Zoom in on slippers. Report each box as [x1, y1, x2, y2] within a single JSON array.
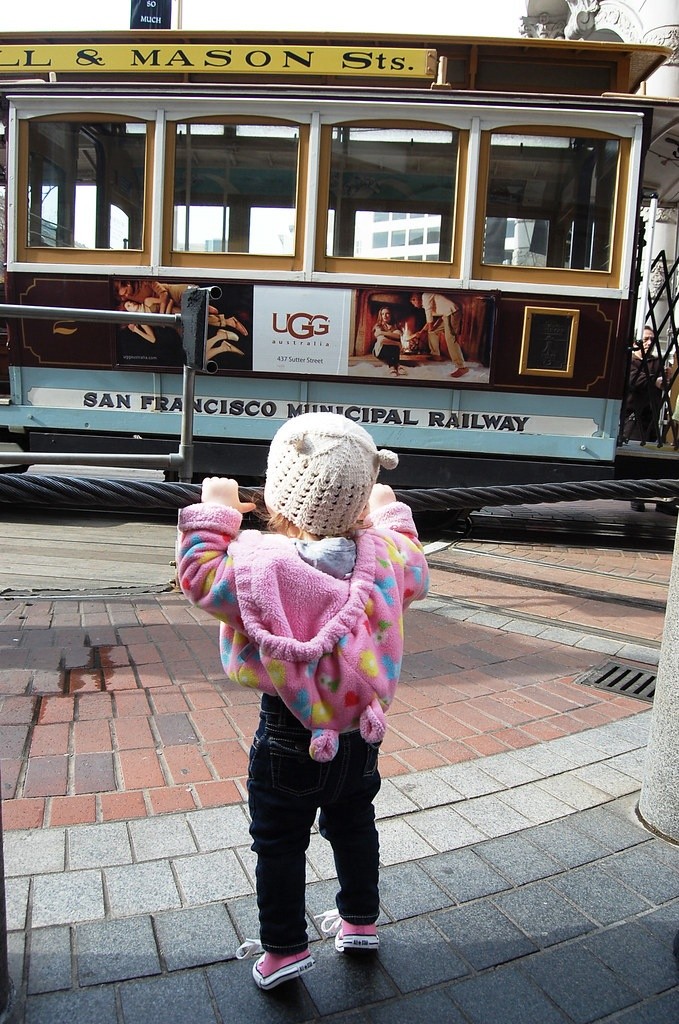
[[232, 317, 248, 336], [398, 365, 406, 375], [389, 369, 397, 377], [208, 305, 218, 314], [218, 329, 239, 341], [223, 340, 244, 356]]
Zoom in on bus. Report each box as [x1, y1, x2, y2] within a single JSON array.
[[0, 30, 679, 537]]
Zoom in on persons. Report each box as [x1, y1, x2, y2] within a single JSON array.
[[408, 290, 470, 379], [170, 407, 431, 992], [370, 304, 408, 377], [114, 279, 220, 317], [115, 295, 246, 357], [616, 324, 668, 444]]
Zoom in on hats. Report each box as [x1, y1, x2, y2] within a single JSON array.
[[264, 411, 399, 536]]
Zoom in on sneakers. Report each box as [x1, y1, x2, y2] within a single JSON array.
[[251, 949, 317, 990], [334, 918, 379, 954]]
